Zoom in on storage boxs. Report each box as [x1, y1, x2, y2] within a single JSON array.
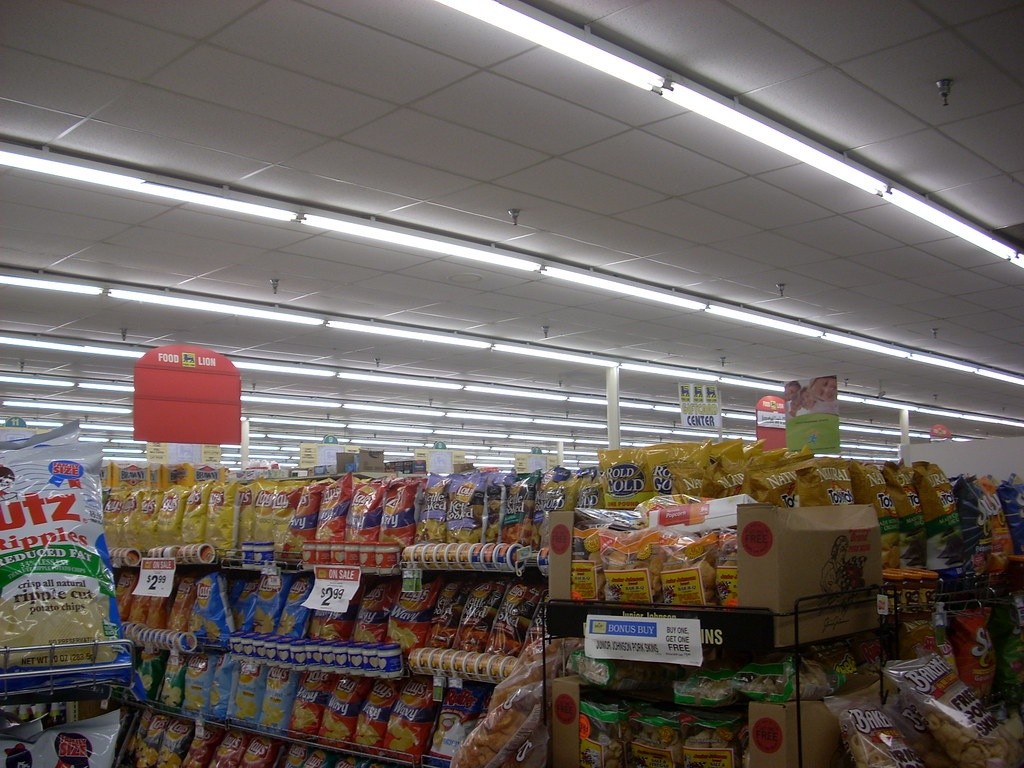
[[551, 674, 883, 768], [548, 503, 883, 648]]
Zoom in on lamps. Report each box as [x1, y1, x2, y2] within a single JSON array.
[[438, 0, 1024, 270], [1, 141, 1024, 466]]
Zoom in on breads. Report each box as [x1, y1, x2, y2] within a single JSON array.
[[582, 546, 737, 607], [449, 685, 527, 768]]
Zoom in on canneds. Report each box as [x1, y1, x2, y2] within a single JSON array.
[[878, 567, 939, 624]]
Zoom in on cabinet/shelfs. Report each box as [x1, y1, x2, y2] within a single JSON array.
[[78, 564, 896, 768]]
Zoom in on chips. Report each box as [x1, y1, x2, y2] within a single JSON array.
[[110, 525, 417, 768]]
